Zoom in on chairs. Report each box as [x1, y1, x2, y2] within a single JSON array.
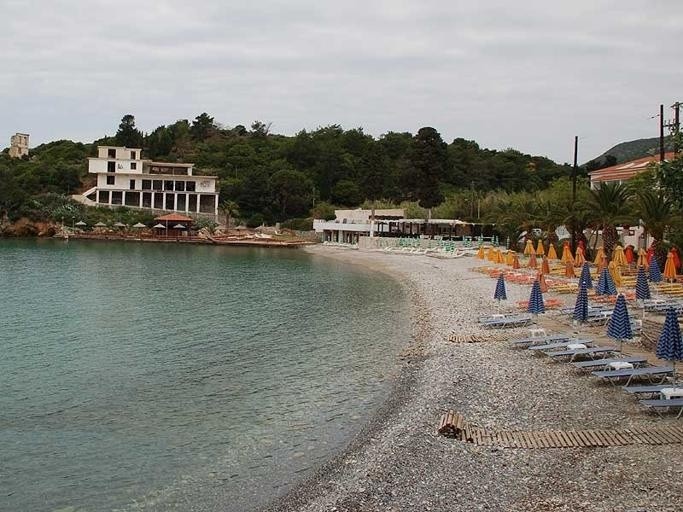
[[479, 296, 683, 420], [470, 257, 683, 348], [382, 245, 517, 259]]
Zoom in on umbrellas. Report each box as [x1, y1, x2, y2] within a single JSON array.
[[606, 293, 635, 361], [152, 222, 167, 239], [173, 223, 187, 238], [572, 279, 590, 345], [112, 221, 127, 237], [656, 303, 683, 390], [75, 221, 87, 226], [133, 221, 147, 239], [473, 234, 683, 323], [94, 221, 107, 232]]
